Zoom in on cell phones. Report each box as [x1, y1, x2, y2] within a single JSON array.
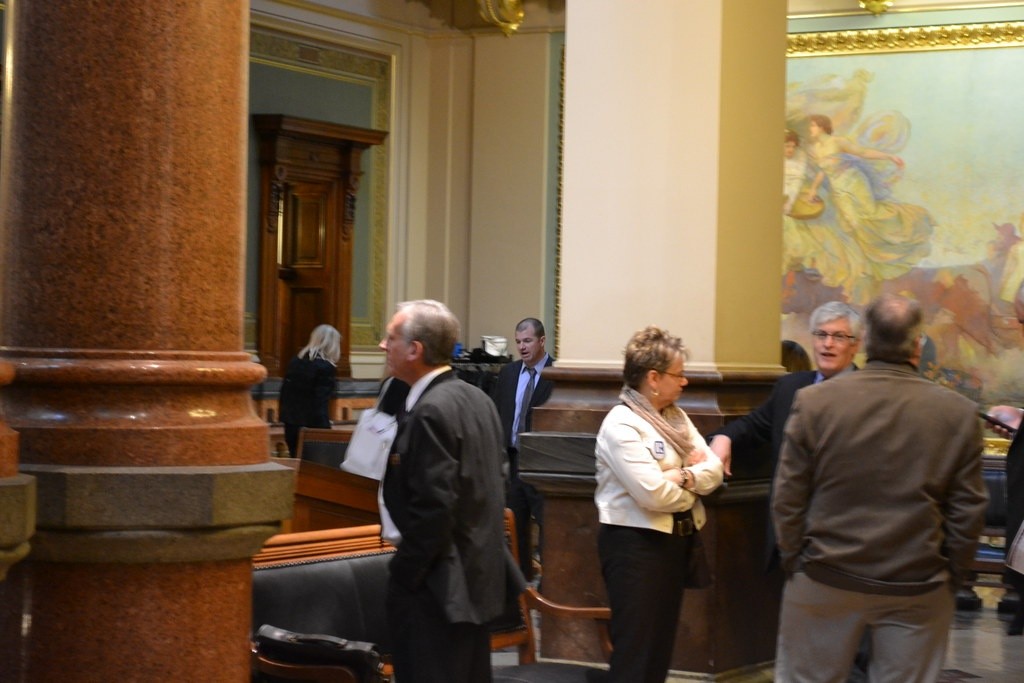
[[977, 412, 1018, 434]]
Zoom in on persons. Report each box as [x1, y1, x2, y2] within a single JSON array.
[[378, 299, 511, 683], [710, 301, 869, 683], [595, 327, 725, 683], [782, 342, 811, 372], [772, 296, 990, 683], [985, 285, 1024, 635], [277, 324, 342, 458], [494, 317, 553, 581]]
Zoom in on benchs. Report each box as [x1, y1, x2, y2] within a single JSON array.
[[959, 455, 1010, 588], [252, 508, 536, 683]]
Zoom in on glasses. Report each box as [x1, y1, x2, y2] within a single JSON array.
[[650, 367, 688, 383], [811, 329, 855, 345]]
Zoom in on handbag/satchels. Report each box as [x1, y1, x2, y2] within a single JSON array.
[[339, 410, 397, 479], [672, 510, 713, 590]]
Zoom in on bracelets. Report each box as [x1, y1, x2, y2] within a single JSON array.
[[675, 467, 689, 487]]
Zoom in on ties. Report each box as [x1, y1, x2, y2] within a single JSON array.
[[517, 368, 538, 435], [396, 405, 407, 427]]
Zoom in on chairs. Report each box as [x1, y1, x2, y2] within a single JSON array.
[[251, 623, 392, 683]]
